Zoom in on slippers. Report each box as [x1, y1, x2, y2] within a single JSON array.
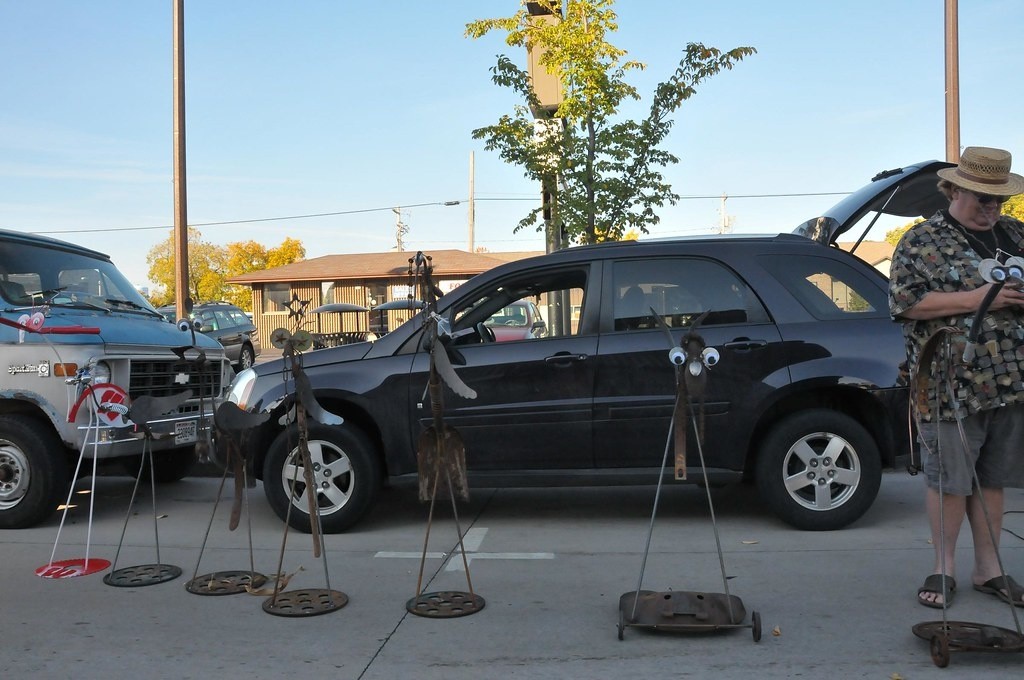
[[974, 575, 1024, 606], [917, 574, 956, 607]]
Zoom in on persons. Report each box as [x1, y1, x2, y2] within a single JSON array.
[[888, 146, 1024, 609]]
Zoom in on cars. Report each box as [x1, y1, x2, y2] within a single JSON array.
[[482, 301, 550, 346]]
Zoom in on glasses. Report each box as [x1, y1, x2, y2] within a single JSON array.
[[953, 188, 1011, 204]]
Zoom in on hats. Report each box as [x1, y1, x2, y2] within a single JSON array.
[[937, 147, 1024, 195]]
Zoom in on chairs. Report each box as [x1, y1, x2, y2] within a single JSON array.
[[616, 285, 646, 331], [0, 279, 32, 304]]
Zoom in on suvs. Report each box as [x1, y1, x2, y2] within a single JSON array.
[[208, 157, 959, 535], [154, 300, 262, 374], [0, 227, 235, 530]]
